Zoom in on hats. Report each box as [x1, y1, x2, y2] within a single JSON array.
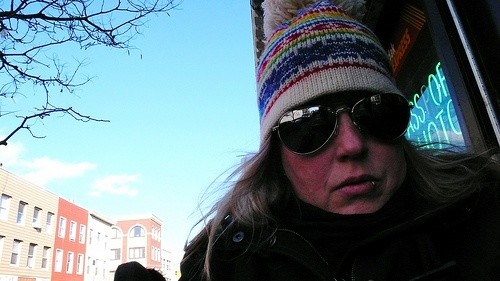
[[258, 0, 410, 150]]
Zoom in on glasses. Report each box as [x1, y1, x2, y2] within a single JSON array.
[[273, 93, 412, 156]]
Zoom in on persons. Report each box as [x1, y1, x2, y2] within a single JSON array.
[[113, 0, 500, 281]]
